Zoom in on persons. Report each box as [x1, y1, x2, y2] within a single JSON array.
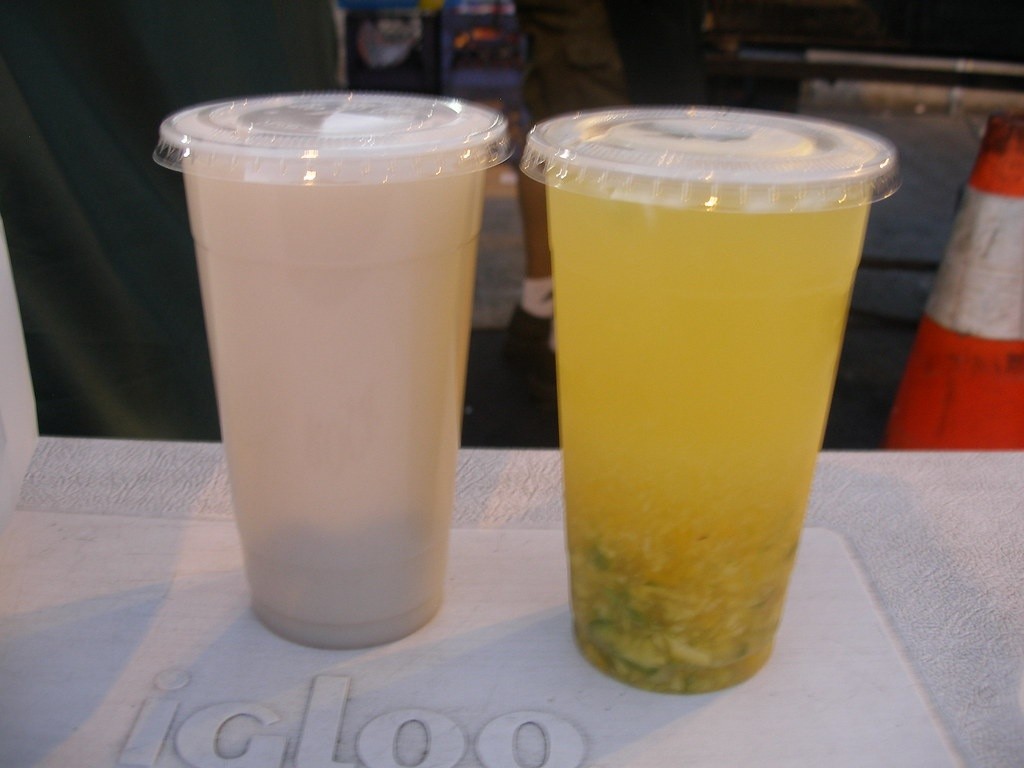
[[502, 0, 706, 397]]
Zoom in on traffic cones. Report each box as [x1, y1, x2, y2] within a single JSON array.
[[886, 109, 1023, 452]]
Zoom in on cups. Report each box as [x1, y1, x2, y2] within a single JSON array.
[[521, 104, 902, 695], [151, 90, 517, 651]]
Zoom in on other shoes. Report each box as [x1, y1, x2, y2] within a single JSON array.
[[503, 303, 555, 407]]
